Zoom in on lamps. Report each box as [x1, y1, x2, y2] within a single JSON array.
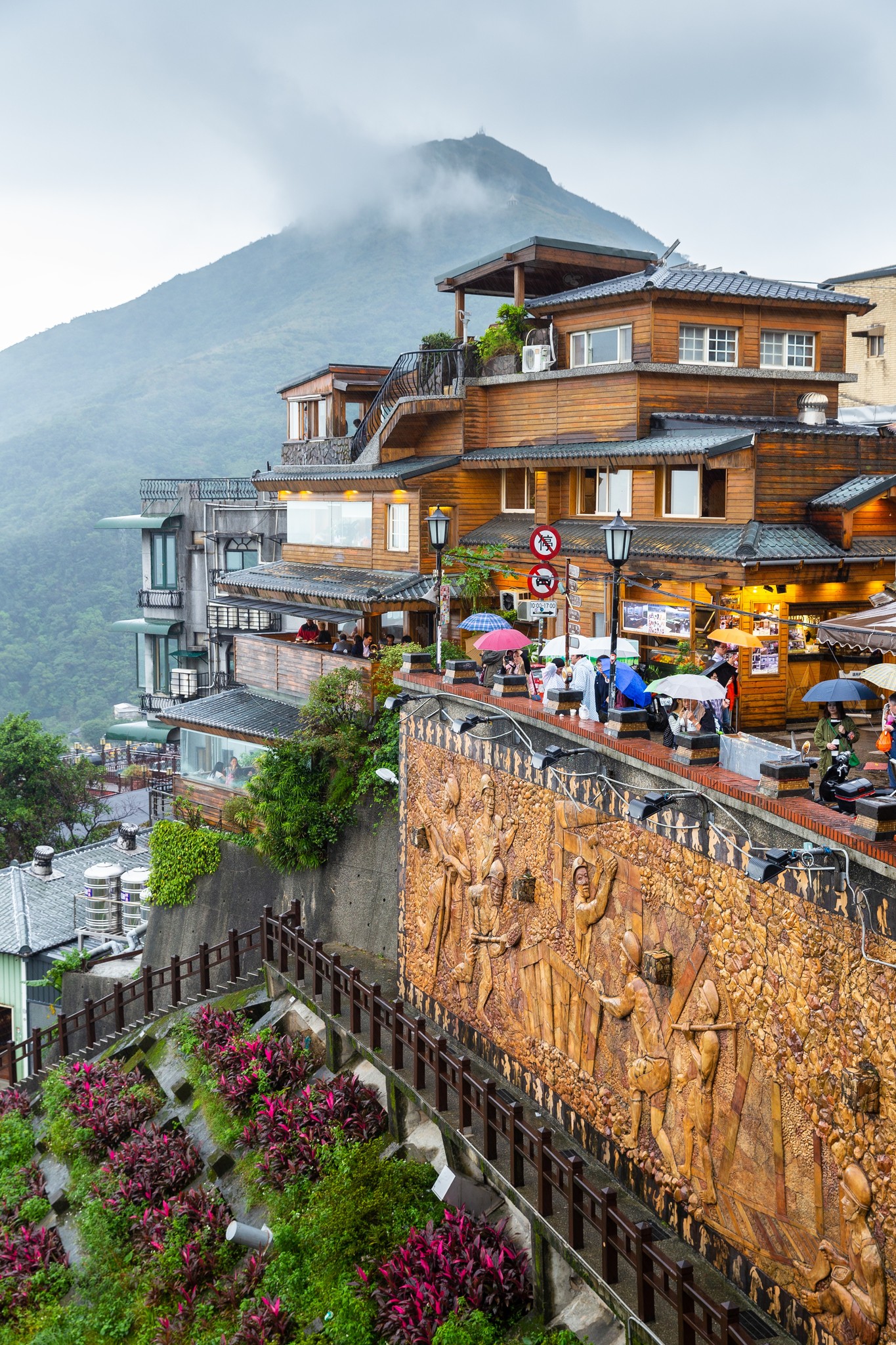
[[529, 743, 607, 782], [626, 788, 713, 834], [739, 844, 833, 883], [381, 690, 448, 722], [450, 711, 523, 745], [652, 580, 663, 591], [762, 585, 775, 594]]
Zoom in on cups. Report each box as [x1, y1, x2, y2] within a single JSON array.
[[299, 638, 303, 640], [721, 621, 726, 628], [804, 650, 808, 653], [886, 715, 895, 725]]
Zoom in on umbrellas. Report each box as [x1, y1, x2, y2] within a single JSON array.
[[706, 628, 765, 654], [643, 677, 666, 694], [539, 634, 595, 666], [587, 634, 640, 659], [599, 658, 651, 708], [455, 611, 513, 635], [464, 633, 485, 667], [654, 674, 728, 712], [472, 625, 534, 655], [698, 661, 739, 689], [859, 663, 896, 720], [801, 673, 880, 725]]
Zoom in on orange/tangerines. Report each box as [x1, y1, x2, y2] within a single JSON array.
[[680, 656, 706, 670]]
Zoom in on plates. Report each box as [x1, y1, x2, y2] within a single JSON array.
[[720, 625, 726, 629]]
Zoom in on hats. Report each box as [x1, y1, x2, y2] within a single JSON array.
[[551, 658, 565, 668]]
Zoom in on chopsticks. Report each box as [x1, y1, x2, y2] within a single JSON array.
[[313, 637, 317, 640]]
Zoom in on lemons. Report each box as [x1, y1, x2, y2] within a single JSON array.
[[659, 655, 673, 663]]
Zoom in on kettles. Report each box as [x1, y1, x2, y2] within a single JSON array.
[[368, 644, 378, 655]]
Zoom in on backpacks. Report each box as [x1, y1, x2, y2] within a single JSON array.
[[705, 707, 723, 735]]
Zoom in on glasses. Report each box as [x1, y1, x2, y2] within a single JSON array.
[[717, 646, 729, 649], [595, 665, 602, 668], [571, 655, 577, 657], [710, 677, 719, 679]]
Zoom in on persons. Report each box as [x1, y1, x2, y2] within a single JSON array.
[[813, 701, 860, 783], [792, 1164, 887, 1345], [296, 618, 412, 659], [883, 691, 896, 788], [481, 649, 633, 725], [663, 641, 736, 751], [206, 756, 254, 785], [412, 773, 720, 1205]]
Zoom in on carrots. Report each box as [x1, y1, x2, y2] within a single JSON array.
[[651, 654, 662, 662]]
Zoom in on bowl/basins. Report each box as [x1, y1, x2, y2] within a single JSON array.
[[570, 709, 576, 716], [806, 644, 819, 652], [580, 710, 589, 719], [296, 639, 299, 642], [286, 641, 291, 642], [302, 640, 307, 642]]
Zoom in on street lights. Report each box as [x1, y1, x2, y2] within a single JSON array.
[[74, 742, 80, 756], [140, 764, 147, 788], [424, 504, 451, 675], [599, 509, 638, 726], [100, 738, 105, 764]]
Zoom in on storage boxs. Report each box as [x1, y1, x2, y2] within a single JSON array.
[[834, 776, 876, 814]]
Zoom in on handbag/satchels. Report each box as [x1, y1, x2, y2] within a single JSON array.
[[844, 751, 860, 767], [479, 670, 485, 683], [530, 694, 542, 702], [876, 729, 893, 752], [663, 725, 674, 747]]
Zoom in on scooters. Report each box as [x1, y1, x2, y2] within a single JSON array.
[[794, 742, 895, 819]]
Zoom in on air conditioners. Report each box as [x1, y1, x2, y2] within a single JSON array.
[[521, 344, 553, 374], [516, 599, 540, 622], [499, 588, 531, 613], [169, 668, 199, 698]]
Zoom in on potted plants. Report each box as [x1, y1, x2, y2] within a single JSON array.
[[122, 763, 152, 789]]
